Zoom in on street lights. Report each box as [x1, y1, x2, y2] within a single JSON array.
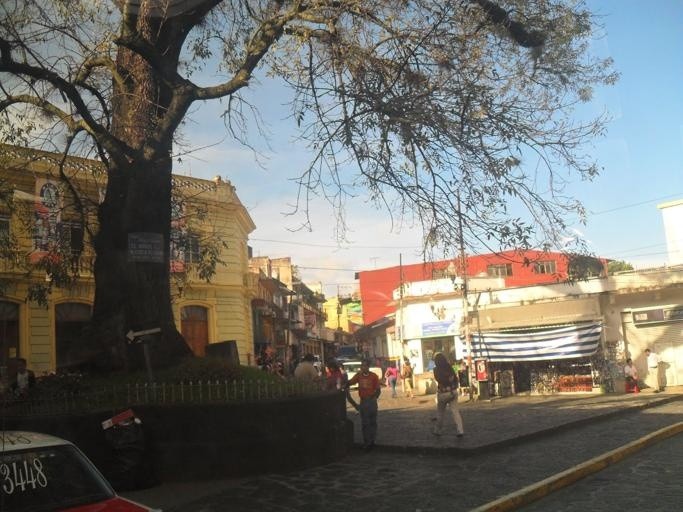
[[448, 268, 474, 405], [283, 290, 296, 376]]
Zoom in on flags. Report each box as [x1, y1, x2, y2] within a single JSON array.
[[29, 176, 66, 267], [169, 196, 189, 275]]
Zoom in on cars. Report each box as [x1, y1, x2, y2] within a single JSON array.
[[298, 354, 320, 373], [0, 427, 158, 511], [335, 355, 363, 391]]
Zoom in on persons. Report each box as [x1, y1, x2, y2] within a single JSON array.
[[623, 356, 641, 392], [254, 351, 360, 419], [399, 360, 416, 399], [428, 351, 464, 438], [340, 357, 381, 454], [384, 359, 398, 398], [642, 347, 664, 392], [7, 358, 36, 397]]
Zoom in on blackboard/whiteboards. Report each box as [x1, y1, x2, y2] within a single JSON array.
[[499, 374, 512, 397]]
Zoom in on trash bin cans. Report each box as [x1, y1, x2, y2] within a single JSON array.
[[479, 380, 490, 400]]
